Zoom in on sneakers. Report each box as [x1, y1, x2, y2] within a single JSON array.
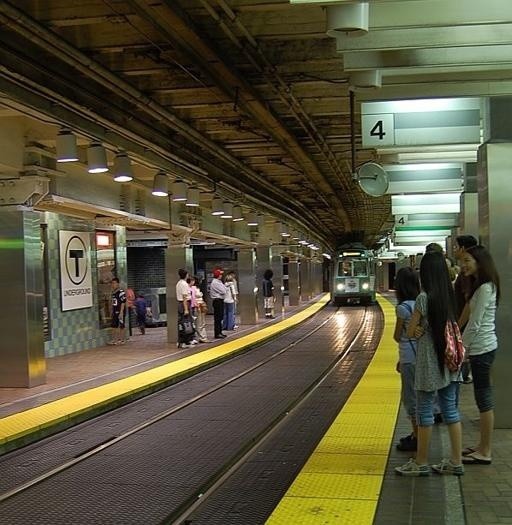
[[394, 458, 430, 477], [176, 337, 211, 348], [397, 431, 417, 451], [233, 325, 240, 329], [431, 458, 466, 475], [108, 338, 127, 345], [265, 314, 275, 319]]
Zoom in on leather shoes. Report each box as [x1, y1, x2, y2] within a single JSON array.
[[214, 332, 227, 338]]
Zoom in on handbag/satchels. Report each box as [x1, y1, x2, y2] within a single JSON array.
[[178, 316, 195, 337]]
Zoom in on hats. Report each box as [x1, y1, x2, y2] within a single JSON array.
[[214, 269, 224, 278]]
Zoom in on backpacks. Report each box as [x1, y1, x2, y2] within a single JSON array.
[[427, 304, 467, 373]]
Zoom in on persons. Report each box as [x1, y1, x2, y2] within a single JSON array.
[[132, 291, 147, 335], [393, 235, 501, 477], [106, 278, 126, 346], [176, 268, 239, 349], [127, 288, 135, 336], [260, 270, 276, 319]]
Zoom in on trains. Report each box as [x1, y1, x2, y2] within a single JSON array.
[[327, 241, 382, 307]]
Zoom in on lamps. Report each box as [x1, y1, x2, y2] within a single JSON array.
[[325, 2, 370, 42], [54, 127, 322, 253], [348, 69, 383, 91]]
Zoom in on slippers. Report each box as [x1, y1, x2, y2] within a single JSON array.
[[462, 444, 492, 465]]
